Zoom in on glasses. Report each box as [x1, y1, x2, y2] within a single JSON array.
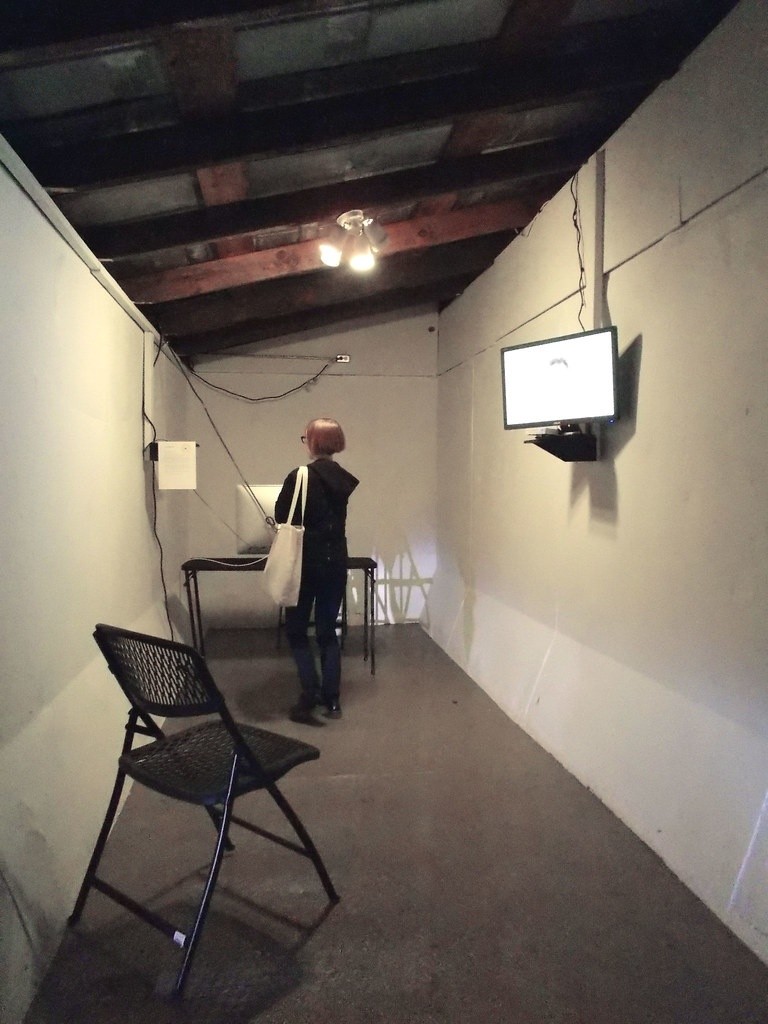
[[301, 436, 307, 443]]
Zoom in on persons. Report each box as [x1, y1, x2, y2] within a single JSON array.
[[259, 418, 359, 720]]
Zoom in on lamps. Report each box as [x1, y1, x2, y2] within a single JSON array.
[[320, 209, 388, 271]]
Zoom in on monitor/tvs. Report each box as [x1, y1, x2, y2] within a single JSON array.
[[501, 325, 619, 429], [237, 484, 285, 555]]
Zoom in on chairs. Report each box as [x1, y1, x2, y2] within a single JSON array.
[[275, 538, 347, 650], [68, 624, 342, 1007]]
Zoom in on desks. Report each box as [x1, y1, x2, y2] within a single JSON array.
[[181, 558, 377, 674]]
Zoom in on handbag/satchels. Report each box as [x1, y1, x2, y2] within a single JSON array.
[[259, 523, 306, 607]]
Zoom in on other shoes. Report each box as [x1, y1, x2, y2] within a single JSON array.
[[290, 689, 324, 714], [322, 695, 341, 714]]
